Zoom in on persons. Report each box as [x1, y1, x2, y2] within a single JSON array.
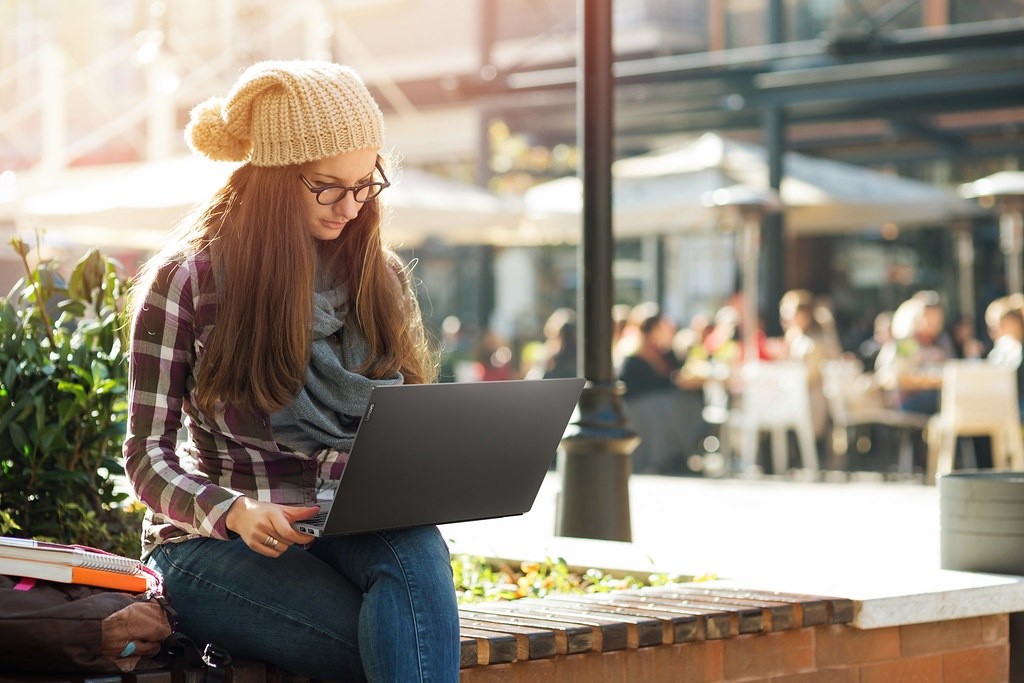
[[522, 286, 1024, 466], [120, 59, 460, 683]]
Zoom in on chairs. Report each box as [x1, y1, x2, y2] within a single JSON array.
[[691, 358, 1023, 485]]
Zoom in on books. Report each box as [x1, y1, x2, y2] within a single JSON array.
[[0, 543, 142, 575], [0, 556, 151, 593]]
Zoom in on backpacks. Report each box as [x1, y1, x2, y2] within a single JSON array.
[[0, 576, 209, 682]]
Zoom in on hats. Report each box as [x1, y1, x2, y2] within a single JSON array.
[[185, 55, 386, 168]]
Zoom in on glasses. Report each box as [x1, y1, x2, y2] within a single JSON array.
[[295, 164, 391, 204]]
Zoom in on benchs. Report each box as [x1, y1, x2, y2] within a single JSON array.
[[79, 579, 855, 683]]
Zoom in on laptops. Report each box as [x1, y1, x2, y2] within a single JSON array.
[[293, 375, 587, 539]]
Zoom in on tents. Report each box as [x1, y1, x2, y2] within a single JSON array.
[[0, 159, 523, 253], [521, 128, 984, 487]]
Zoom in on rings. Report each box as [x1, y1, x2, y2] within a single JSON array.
[[264, 536, 278, 549]]
